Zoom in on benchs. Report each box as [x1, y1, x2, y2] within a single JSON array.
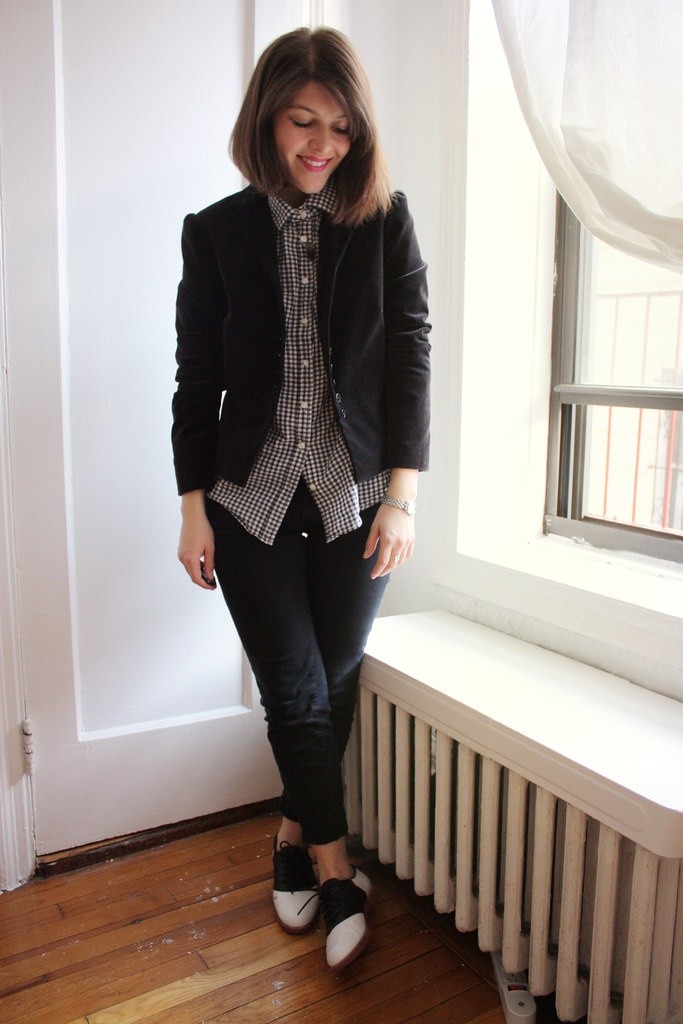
[[357, 610, 683, 856]]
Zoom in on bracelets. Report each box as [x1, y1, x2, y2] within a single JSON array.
[[381, 496, 416, 516]]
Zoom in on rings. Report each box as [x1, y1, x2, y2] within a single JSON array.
[[393, 553, 400, 566]]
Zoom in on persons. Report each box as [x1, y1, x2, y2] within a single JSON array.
[[170, 25, 433, 972]]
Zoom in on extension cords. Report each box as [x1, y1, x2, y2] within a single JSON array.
[[491, 950, 538, 1024]]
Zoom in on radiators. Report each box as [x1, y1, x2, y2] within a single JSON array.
[[341, 684, 683, 1024]]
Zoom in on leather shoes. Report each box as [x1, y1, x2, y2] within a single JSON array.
[[272, 830, 321, 935], [318, 863, 376, 973]]
